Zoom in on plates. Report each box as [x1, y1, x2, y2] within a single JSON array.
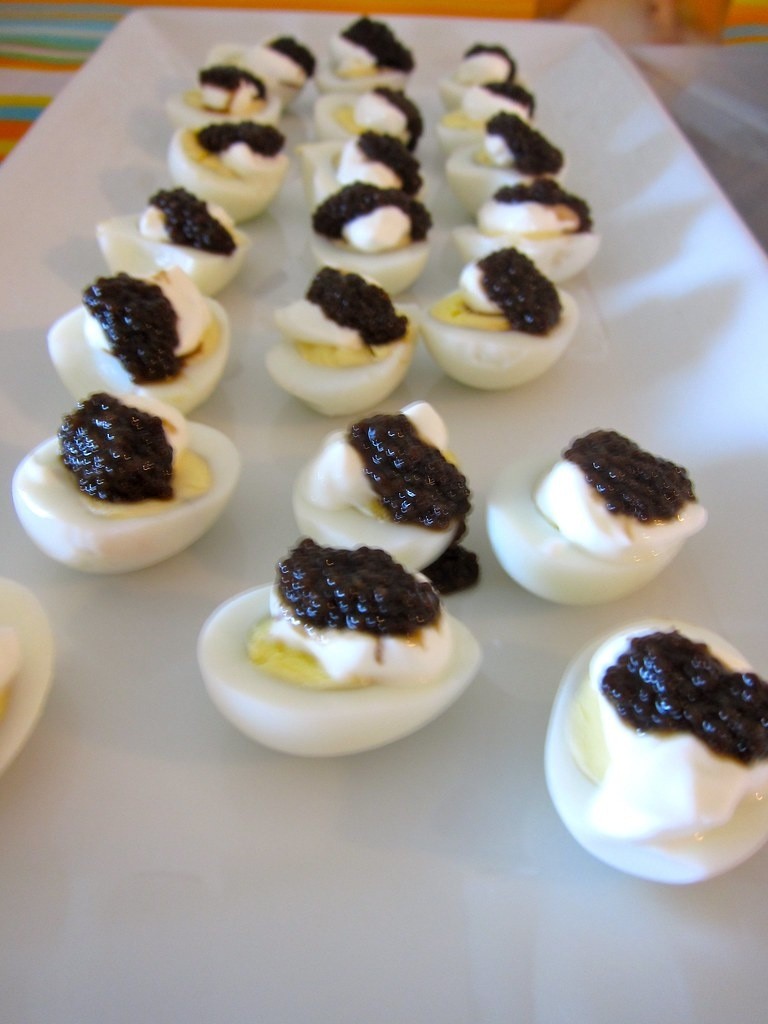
[[0, 7, 768, 1024]]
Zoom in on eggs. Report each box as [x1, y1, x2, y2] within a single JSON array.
[[0, 15, 768, 886]]
